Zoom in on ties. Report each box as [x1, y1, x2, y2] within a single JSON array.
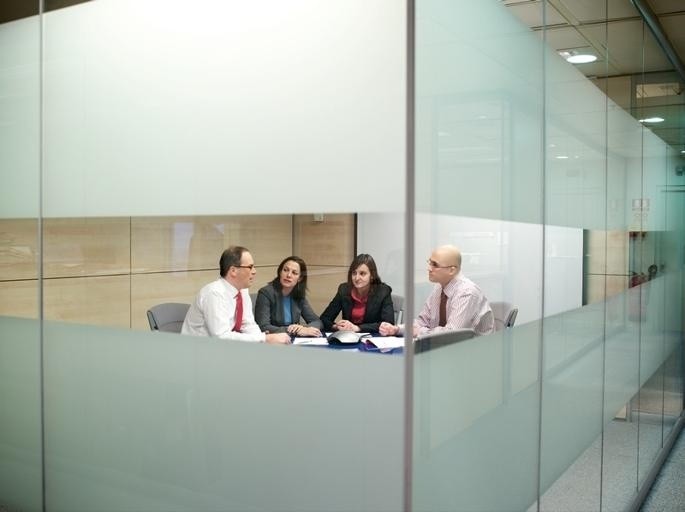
[[232, 292, 243, 332], [439, 292, 448, 327]]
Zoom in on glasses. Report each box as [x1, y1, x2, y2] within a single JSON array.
[[427, 258, 456, 270], [236, 265, 254, 270]]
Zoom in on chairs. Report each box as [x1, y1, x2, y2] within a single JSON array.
[[147, 302, 193, 332], [486, 299, 518, 331]]
